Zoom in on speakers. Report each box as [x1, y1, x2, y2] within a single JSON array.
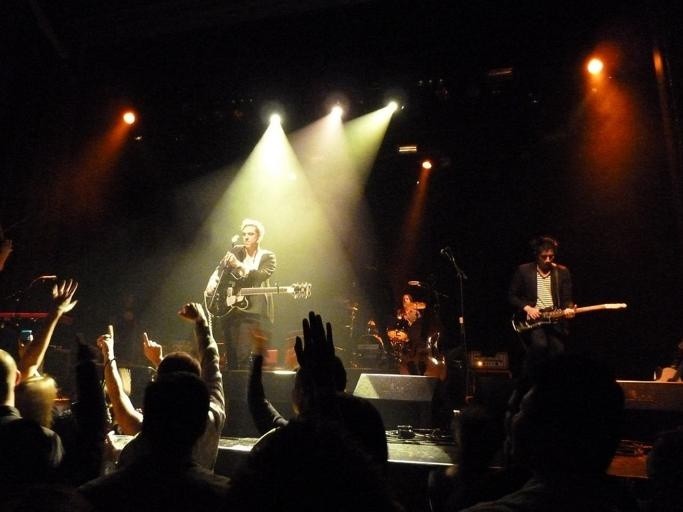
[[221, 370, 297, 437], [353, 373, 443, 427], [617, 378, 683, 444]]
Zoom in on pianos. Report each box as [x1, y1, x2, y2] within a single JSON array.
[[1, 275, 72, 325]]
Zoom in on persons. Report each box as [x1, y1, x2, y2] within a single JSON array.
[[204, 218, 278, 373], [508, 237, 578, 383], [0, 240, 14, 271]]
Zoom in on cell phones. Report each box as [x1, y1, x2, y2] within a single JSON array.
[[19, 329, 33, 349]]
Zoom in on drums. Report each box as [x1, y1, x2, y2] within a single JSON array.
[[357, 335, 384, 367]]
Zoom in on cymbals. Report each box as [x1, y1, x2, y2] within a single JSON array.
[[388, 331, 406, 339]]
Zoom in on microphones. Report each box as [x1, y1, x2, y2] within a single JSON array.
[[440, 247, 455, 264], [232, 235, 240, 244]]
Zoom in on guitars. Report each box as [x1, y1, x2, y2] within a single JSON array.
[[205, 271, 312, 318], [512, 302, 627, 331]]
